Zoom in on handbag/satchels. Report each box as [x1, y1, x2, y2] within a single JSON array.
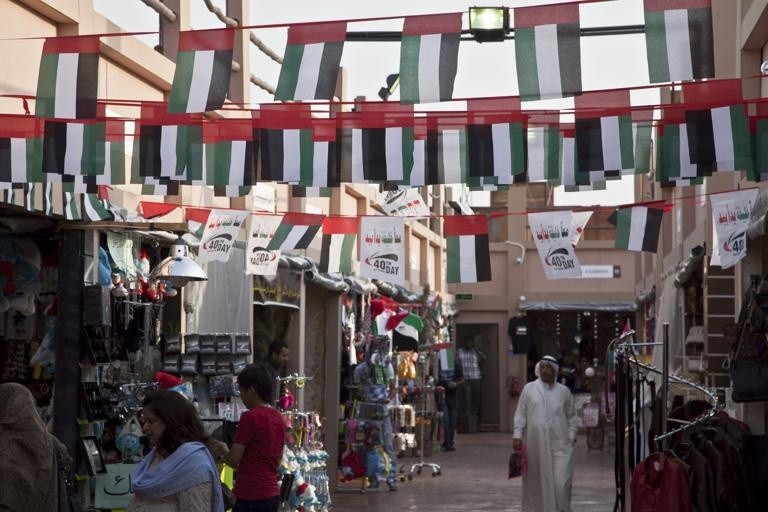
[[507, 444, 530, 478]]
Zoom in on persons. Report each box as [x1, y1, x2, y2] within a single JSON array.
[[0, 382, 81, 512], [428, 338, 466, 453], [126, 390, 224, 512], [455, 335, 487, 433], [556, 351, 578, 394], [215, 363, 285, 512], [513, 354, 579, 512], [352, 349, 401, 493], [260, 340, 289, 379]]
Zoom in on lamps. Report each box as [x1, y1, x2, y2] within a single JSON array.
[[377, 73, 399, 100], [147, 232, 209, 289], [469, 6, 510, 43]]
